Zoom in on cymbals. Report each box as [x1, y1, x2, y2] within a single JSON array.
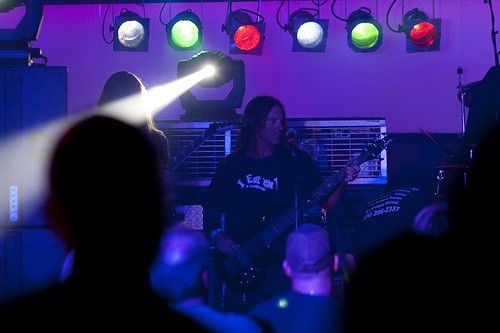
[[433, 164, 485, 175], [457, 80, 482, 109]]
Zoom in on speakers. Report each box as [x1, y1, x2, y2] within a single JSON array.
[[0, 64, 70, 228]]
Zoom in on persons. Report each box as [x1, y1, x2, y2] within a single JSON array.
[[96, 70, 176, 218], [242, 224, 339, 333], [0, 114, 218, 333], [150, 230, 261, 333], [202, 95, 362, 313], [357, 175, 454, 241], [323, 111, 500, 333]]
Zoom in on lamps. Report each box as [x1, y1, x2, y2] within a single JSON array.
[[275, 0, 330, 54], [101, 1, 150, 53], [159, 2, 203, 51], [221, 0, 267, 56], [331, 0, 383, 54], [176, 50, 247, 116], [386, 0, 442, 54]]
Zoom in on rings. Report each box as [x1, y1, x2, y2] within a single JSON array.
[[353, 174, 356, 178]]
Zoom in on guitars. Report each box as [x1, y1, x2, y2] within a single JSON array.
[[206, 132, 395, 291], [166, 119, 248, 172]]
